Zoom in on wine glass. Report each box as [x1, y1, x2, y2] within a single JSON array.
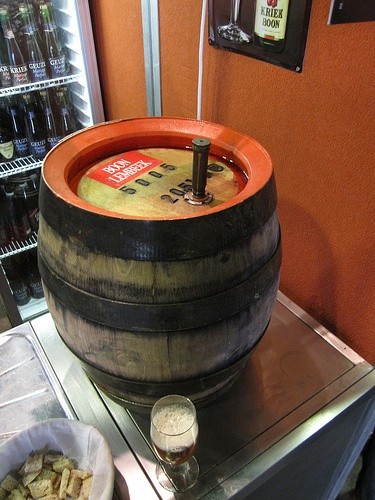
[[217, 0, 252, 45], [150, 395, 199, 493]]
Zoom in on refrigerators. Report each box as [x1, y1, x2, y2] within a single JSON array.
[[0, 0, 104, 328]]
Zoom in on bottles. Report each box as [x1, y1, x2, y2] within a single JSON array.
[[0, 0, 70, 88], [253, 0, 290, 53], [0, 248, 44, 306], [0, 167, 42, 248], [0, 85, 76, 161]]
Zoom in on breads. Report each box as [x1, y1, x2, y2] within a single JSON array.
[[0, 448, 93, 500]]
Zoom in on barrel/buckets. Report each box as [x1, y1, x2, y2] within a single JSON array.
[[38, 116, 283, 414]]
